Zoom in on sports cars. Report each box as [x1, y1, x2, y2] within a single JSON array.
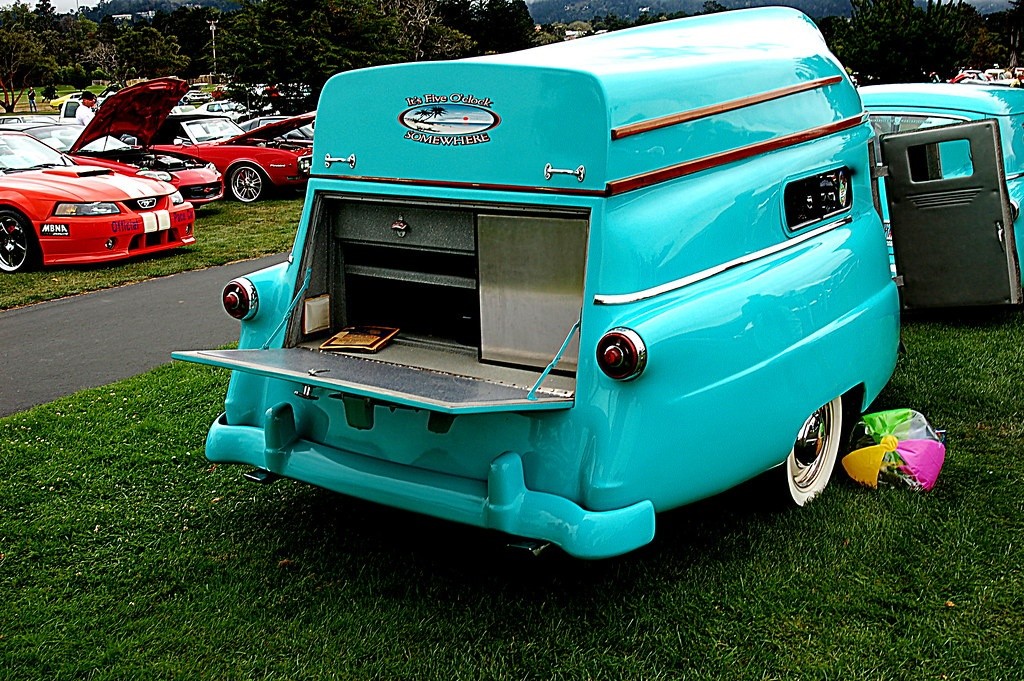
[[0, 76, 196, 275]]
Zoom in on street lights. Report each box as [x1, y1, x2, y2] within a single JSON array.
[[206, 18, 220, 84]]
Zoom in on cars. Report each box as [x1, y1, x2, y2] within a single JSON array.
[[0, 83, 317, 210], [852, 82, 1024, 291], [168, 1, 1022, 561]]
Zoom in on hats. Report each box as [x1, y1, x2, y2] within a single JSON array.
[[79, 91, 94, 100]]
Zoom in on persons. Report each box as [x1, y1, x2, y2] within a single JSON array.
[[76, 91, 101, 126], [40, 81, 59, 103], [1002, 64, 1023, 88], [27, 86, 38, 113]]
